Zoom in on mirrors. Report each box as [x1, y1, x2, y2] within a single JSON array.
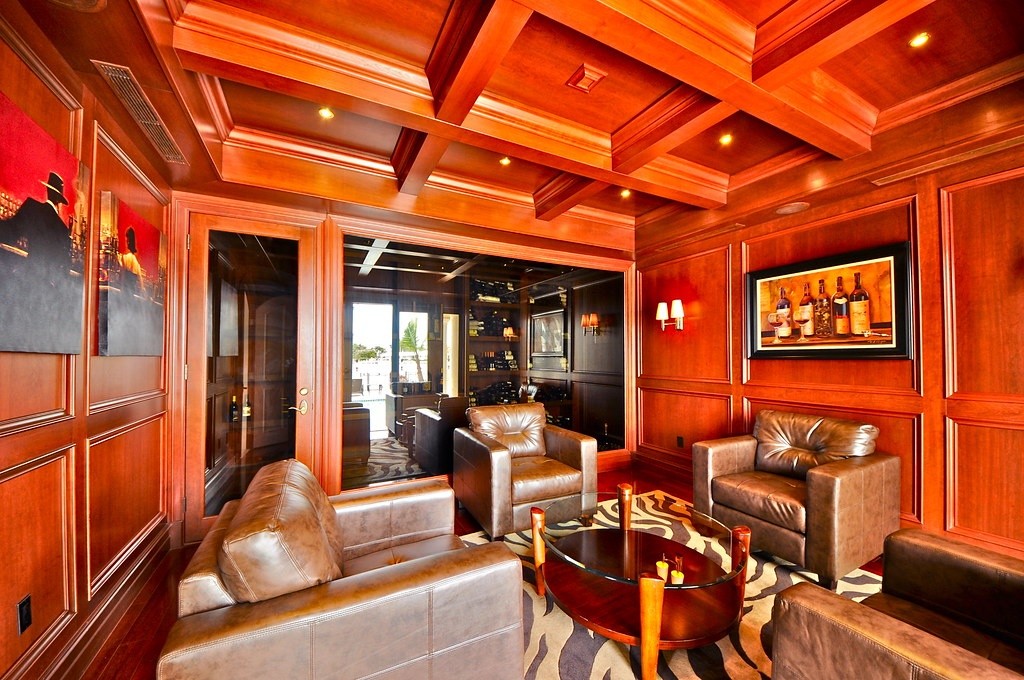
[[342, 232, 626, 492]]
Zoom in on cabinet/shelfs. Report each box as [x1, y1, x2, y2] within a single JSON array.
[[453, 275, 526, 404]]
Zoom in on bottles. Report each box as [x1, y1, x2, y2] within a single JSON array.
[[850, 272, 870, 336], [546, 410, 572, 430], [799, 282, 815, 337], [813, 279, 832, 338], [247, 394, 252, 420], [776, 287, 792, 338], [230, 396, 239, 422], [831, 276, 851, 338], [468, 350, 519, 372], [469, 381, 520, 406], [469, 308, 518, 337], [603, 423, 611, 450], [534, 384, 568, 401], [437, 368, 443, 393], [470, 278, 519, 304]]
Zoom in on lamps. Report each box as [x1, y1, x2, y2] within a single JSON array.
[[656, 300, 684, 330], [581, 314, 598, 336]]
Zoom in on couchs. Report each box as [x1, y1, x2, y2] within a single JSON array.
[[156, 458, 524, 680], [518, 382, 560, 423], [771, 527, 1024, 680], [385, 383, 449, 440], [692, 410, 900, 590], [343, 401, 371, 469], [452, 402, 597, 542], [414, 397, 469, 475]]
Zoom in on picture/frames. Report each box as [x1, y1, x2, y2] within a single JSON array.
[[747, 240, 914, 360], [530, 309, 566, 357]]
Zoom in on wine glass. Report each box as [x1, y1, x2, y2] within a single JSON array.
[[794, 310, 810, 343], [768, 313, 785, 344]]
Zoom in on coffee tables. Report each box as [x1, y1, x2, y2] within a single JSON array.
[[530, 484, 751, 680]]
